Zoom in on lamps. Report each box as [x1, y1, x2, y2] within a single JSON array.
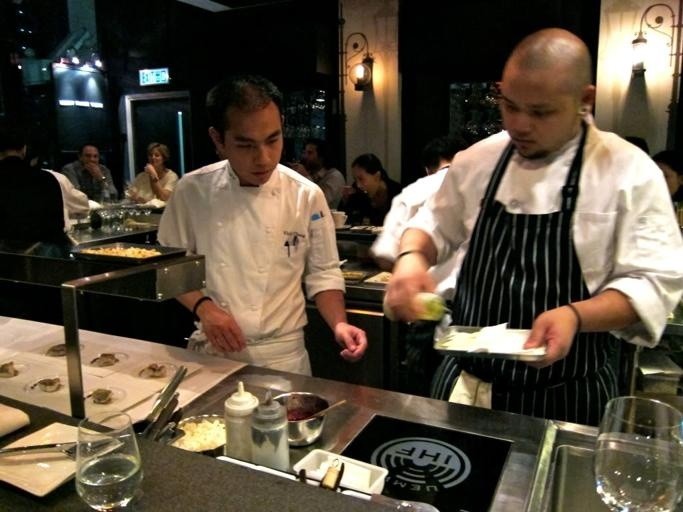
[[315, 88, 325, 103], [341, 32, 375, 92], [629, 4, 675, 74]]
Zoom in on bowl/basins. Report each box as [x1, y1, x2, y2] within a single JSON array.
[[167, 412, 229, 459], [267, 390, 328, 449]]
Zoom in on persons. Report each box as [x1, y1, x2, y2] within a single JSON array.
[[0, 124, 176, 252], [155, 69, 368, 379], [289, 137, 683, 231], [381, 27, 683, 433]]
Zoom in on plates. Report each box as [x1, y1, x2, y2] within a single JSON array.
[[0, 419, 126, 496]]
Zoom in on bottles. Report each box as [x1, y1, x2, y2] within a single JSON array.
[[275, 86, 330, 140], [381, 286, 452, 323], [222, 378, 261, 464], [250, 391, 293, 471]]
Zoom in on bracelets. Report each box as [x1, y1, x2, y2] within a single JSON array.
[[395, 249, 435, 269], [191, 293, 213, 317], [566, 303, 582, 336]]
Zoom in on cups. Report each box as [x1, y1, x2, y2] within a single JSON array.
[[75, 414, 146, 510], [594, 395, 683, 512], [330, 209, 347, 229], [62, 176, 154, 228]]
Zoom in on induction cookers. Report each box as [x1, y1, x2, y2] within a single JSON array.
[[336, 413, 514, 511]]
